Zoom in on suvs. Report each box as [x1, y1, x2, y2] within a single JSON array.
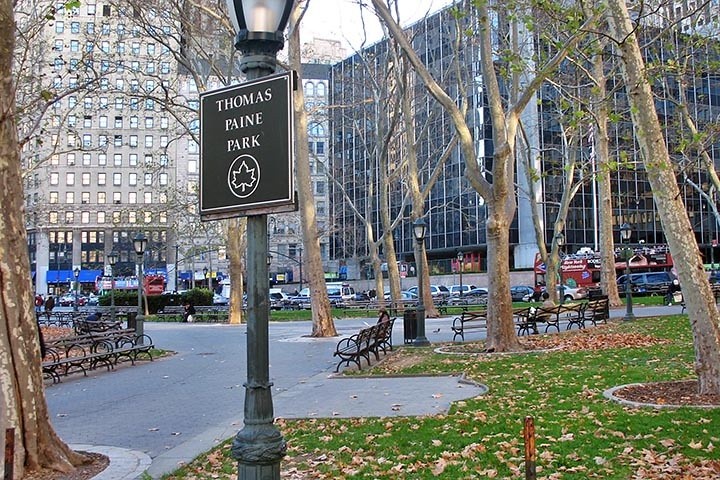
[[616, 271, 674, 299], [268, 288, 288, 311]]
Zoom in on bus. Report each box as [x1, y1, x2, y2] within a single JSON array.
[[93, 274, 165, 296], [533, 252, 673, 287]]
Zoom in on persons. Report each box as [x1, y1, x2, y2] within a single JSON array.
[[35, 294, 55, 319], [528, 293, 555, 334], [666, 278, 681, 306], [363, 288, 376, 298], [533, 285, 542, 302], [86, 312, 102, 327], [182, 303, 196, 322], [371, 308, 390, 339]]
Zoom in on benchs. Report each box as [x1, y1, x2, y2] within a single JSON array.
[[102, 310, 127, 323], [41, 329, 155, 384], [333, 318, 396, 372], [343, 298, 488, 318], [580, 299, 609, 327], [681, 286, 720, 314], [529, 303, 583, 332], [159, 306, 203, 323], [38, 311, 92, 328], [450, 307, 531, 342], [207, 306, 246, 324], [72, 319, 122, 336]]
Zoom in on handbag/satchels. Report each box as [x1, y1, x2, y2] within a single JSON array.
[[187, 315, 193, 322]]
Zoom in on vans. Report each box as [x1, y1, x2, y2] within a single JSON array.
[[289, 281, 355, 311]]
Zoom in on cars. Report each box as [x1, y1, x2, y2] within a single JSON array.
[[510, 286, 534, 302], [522, 283, 589, 303], [160, 290, 191, 295], [36, 292, 100, 307], [212, 294, 228, 306], [707, 274, 720, 298], [355, 284, 490, 310]]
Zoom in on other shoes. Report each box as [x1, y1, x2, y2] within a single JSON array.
[[668, 303, 672, 307], [531, 331, 539, 334]]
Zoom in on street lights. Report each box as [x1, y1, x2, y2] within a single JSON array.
[[456, 251, 464, 297], [411, 214, 431, 347], [224, 1, 300, 480], [554, 231, 565, 304], [132, 229, 148, 344], [107, 251, 118, 322], [203, 266, 208, 289], [266, 253, 274, 289], [619, 222, 637, 322], [73, 266, 81, 311]]
